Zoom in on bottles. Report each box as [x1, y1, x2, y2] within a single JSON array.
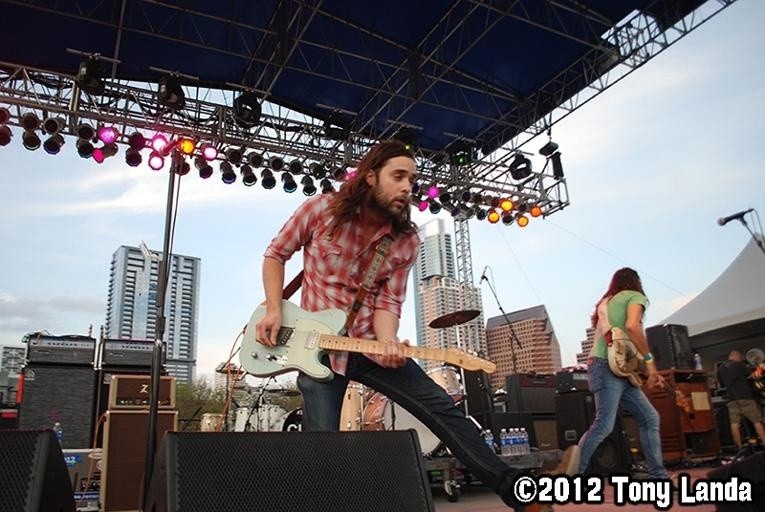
[[693, 353, 703, 370], [52, 421, 64, 450], [484, 427, 531, 457]]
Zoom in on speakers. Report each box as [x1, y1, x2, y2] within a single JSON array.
[[95, 367, 168, 445], [13, 366, 97, 448], [0, 428, 79, 511], [144, 427, 438, 511], [644, 323, 696, 369], [101, 412, 178, 512], [554, 393, 617, 448], [466, 368, 491, 412], [591, 436, 635, 477], [470, 413, 490, 430], [489, 414, 538, 448]]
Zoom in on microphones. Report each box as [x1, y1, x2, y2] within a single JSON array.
[[479, 266, 488, 288], [717, 207, 754, 225]]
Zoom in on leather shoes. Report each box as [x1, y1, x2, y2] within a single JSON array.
[[515, 445, 581, 512]]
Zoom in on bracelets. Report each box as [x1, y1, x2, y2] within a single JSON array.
[[643, 351, 655, 365]]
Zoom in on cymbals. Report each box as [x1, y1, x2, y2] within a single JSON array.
[[267, 389, 300, 397], [217, 370, 250, 374], [429, 310, 480, 329]]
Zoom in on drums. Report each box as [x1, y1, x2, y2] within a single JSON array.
[[425, 366, 465, 406], [364, 392, 441, 458], [200, 413, 225, 432], [340, 385, 375, 432], [234, 405, 303, 433]]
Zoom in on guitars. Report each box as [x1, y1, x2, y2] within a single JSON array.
[[608, 327, 691, 414], [239, 300, 498, 383]]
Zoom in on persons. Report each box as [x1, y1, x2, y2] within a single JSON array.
[[579, 265, 675, 493], [255, 138, 586, 510], [716, 350, 765, 450]]
